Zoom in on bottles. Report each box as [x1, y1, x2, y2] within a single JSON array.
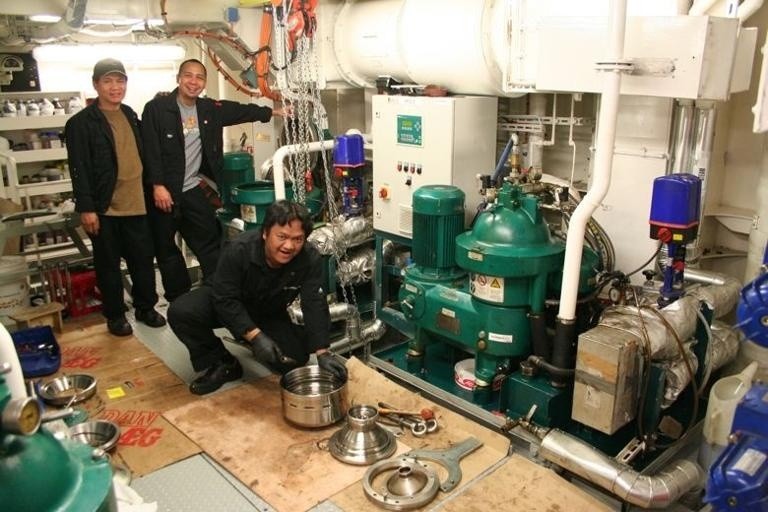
[[2, 96, 80, 117], [28, 129, 68, 149]]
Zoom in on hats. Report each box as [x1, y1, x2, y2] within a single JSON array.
[[94, 58, 127, 81]]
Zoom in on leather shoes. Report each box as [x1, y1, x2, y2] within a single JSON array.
[[108, 312, 133, 335], [190, 357, 242, 394], [135, 309, 165, 327]]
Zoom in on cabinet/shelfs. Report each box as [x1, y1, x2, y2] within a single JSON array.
[[0, 89, 93, 263]]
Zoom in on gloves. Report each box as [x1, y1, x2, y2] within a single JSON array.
[[250, 332, 282, 364], [318, 351, 348, 380]]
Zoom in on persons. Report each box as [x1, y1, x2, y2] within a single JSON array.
[[140, 58, 293, 302], [64, 58, 167, 337], [166, 199, 348, 395]]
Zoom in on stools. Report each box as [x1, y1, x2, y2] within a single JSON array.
[[49, 270, 104, 316], [7, 301, 64, 334]]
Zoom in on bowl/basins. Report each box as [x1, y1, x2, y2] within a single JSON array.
[[37, 372, 98, 407], [52, 419, 122, 458]]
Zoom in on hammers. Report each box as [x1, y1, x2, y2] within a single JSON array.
[[377, 408, 435, 420]]
